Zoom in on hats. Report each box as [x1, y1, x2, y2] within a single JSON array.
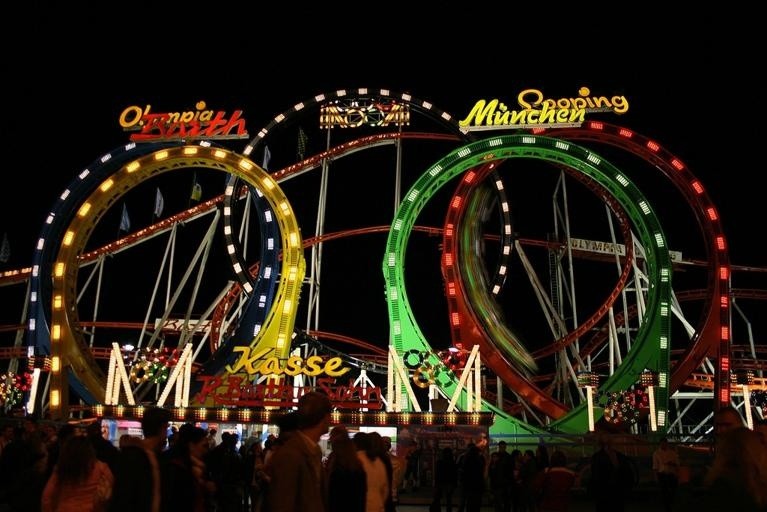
[[247, 436, 261, 449]]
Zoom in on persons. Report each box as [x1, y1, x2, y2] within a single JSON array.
[[0, 375, 395, 510], [402, 440, 419, 491], [429, 405, 766, 510]]
